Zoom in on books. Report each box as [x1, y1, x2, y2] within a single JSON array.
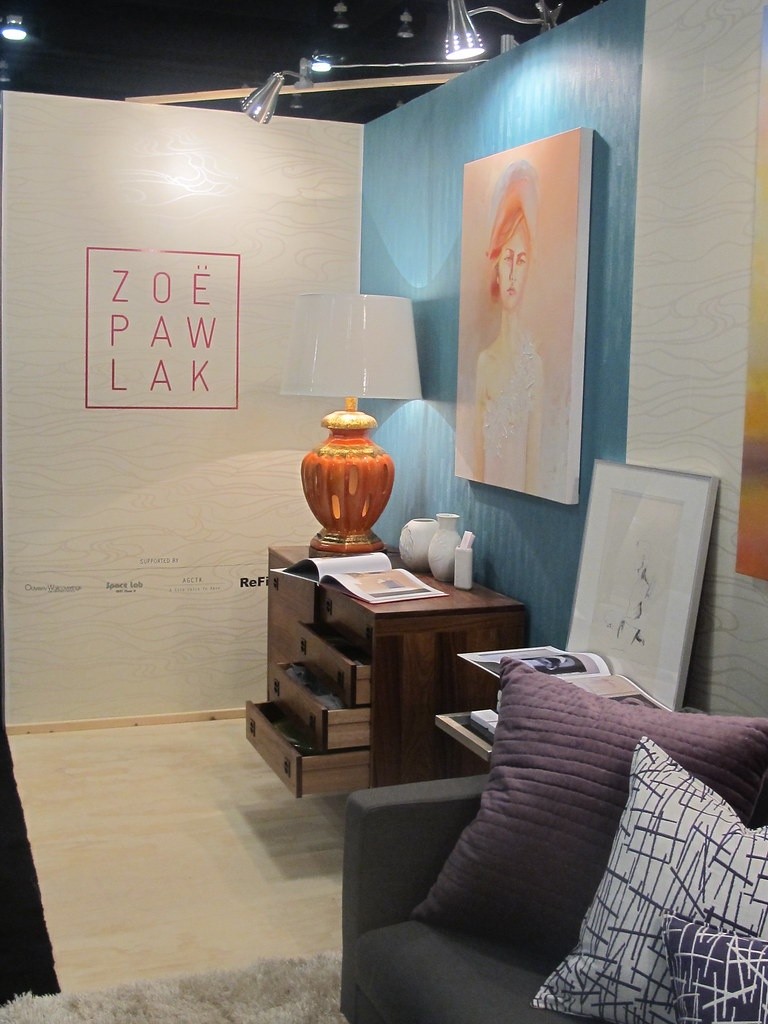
[[270, 551, 450, 604], [456, 642, 673, 712]]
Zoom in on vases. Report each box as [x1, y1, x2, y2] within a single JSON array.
[[398, 512, 463, 583]]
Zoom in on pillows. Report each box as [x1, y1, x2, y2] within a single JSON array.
[[527, 734, 768, 1024], [405, 655, 768, 963], [660, 915, 768, 1024]]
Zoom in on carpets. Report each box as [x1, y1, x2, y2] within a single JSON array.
[[0, 952, 348, 1024]]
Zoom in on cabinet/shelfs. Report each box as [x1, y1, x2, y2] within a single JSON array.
[[245, 544, 532, 799]]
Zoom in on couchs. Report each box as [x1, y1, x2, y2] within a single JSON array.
[[340, 772, 768, 1024]]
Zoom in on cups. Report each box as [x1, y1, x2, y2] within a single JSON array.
[[455, 546, 473, 589]]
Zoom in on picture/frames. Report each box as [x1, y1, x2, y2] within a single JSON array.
[[564, 459, 721, 713]]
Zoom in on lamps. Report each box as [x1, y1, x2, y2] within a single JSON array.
[[1, 14, 28, 41], [240, 58, 312, 124], [332, 3, 349, 29], [278, 293, 423, 558], [310, 54, 490, 73], [443, 0, 563, 62], [396, 11, 415, 38]]
